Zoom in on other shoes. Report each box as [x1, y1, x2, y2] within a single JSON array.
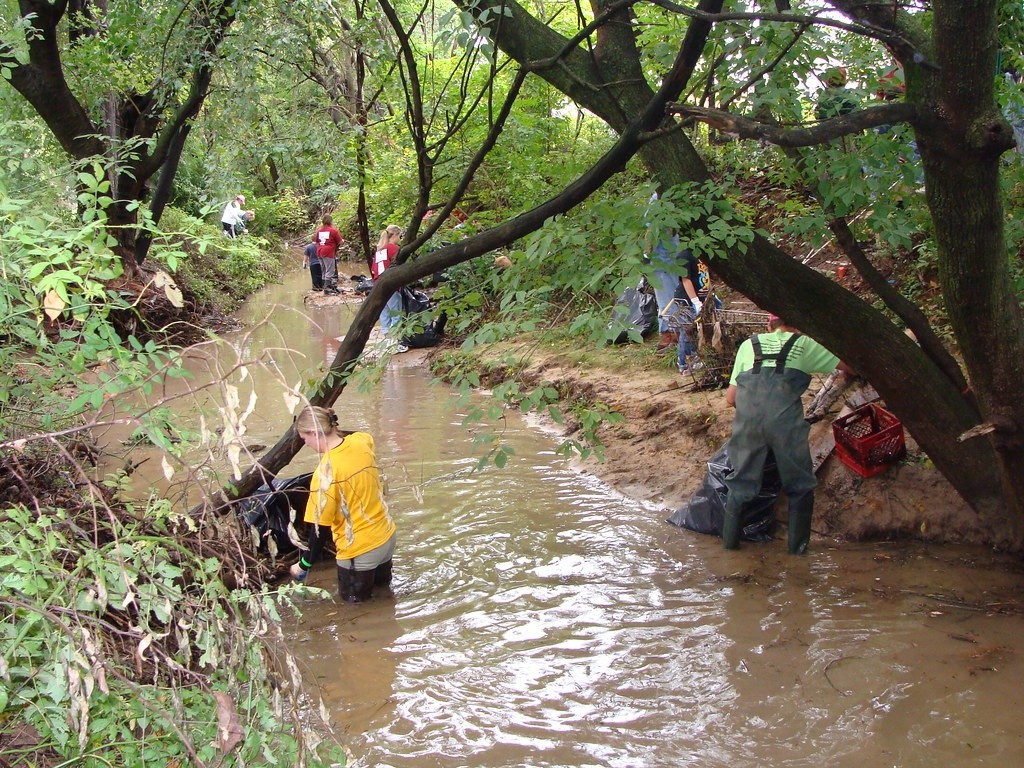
[[680, 366, 695, 376], [690, 362, 703, 373]]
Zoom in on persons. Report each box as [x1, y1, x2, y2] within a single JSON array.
[[674, 235, 723, 376], [302, 235, 323, 292], [236, 209, 256, 235], [640, 190, 685, 360], [999, 43, 1024, 167], [722, 310, 919, 555], [812, 54, 927, 217], [315, 214, 344, 295], [222, 194, 246, 239], [420, 182, 513, 339], [368, 224, 409, 356], [289, 404, 401, 605]]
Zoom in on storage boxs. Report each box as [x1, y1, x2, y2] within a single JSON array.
[[831, 402, 906, 478]]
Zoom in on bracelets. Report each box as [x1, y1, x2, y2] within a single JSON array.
[[301, 557, 312, 568]]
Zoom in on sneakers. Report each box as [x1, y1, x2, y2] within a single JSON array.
[[386, 341, 398, 353], [391, 344, 408, 355]]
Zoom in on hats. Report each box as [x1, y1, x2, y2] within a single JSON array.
[[237, 195, 245, 204]]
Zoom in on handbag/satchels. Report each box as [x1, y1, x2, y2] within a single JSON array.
[[229, 473, 333, 553], [666, 439, 783, 544], [402, 289, 440, 347], [607, 281, 657, 343]]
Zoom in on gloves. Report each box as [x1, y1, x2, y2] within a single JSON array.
[[303, 262, 307, 269], [692, 297, 702, 315], [289, 561, 308, 581]]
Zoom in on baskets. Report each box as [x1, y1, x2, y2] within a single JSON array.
[[832, 403, 906, 477]]
